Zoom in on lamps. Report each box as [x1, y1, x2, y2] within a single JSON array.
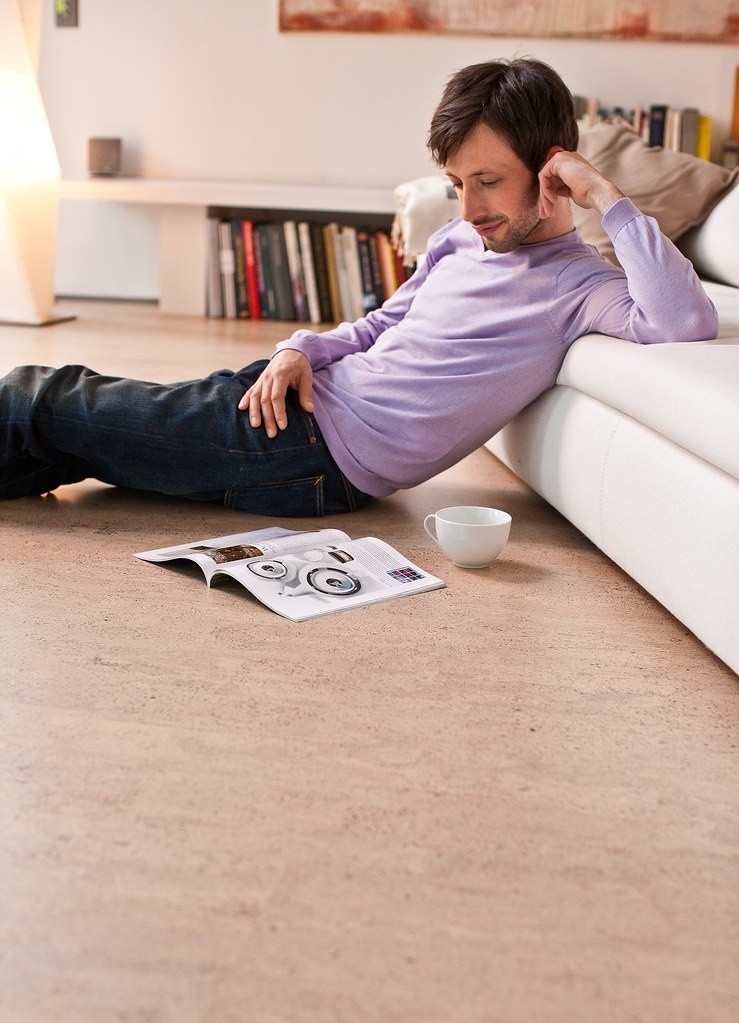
[[0, 0, 77, 328]]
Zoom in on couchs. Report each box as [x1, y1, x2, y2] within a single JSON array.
[[388, 174, 739, 682]]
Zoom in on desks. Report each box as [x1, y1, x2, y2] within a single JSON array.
[[81, 177, 397, 318]]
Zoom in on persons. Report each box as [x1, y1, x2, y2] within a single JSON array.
[[0, 58, 719, 516]]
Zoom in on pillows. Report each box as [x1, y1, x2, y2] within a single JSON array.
[[568, 121, 739, 271]]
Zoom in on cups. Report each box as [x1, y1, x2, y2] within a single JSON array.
[[423, 505, 512, 569]]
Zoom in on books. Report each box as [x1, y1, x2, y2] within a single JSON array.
[[132, 525, 446, 624], [572, 96, 712, 163], [207, 217, 417, 323]]
